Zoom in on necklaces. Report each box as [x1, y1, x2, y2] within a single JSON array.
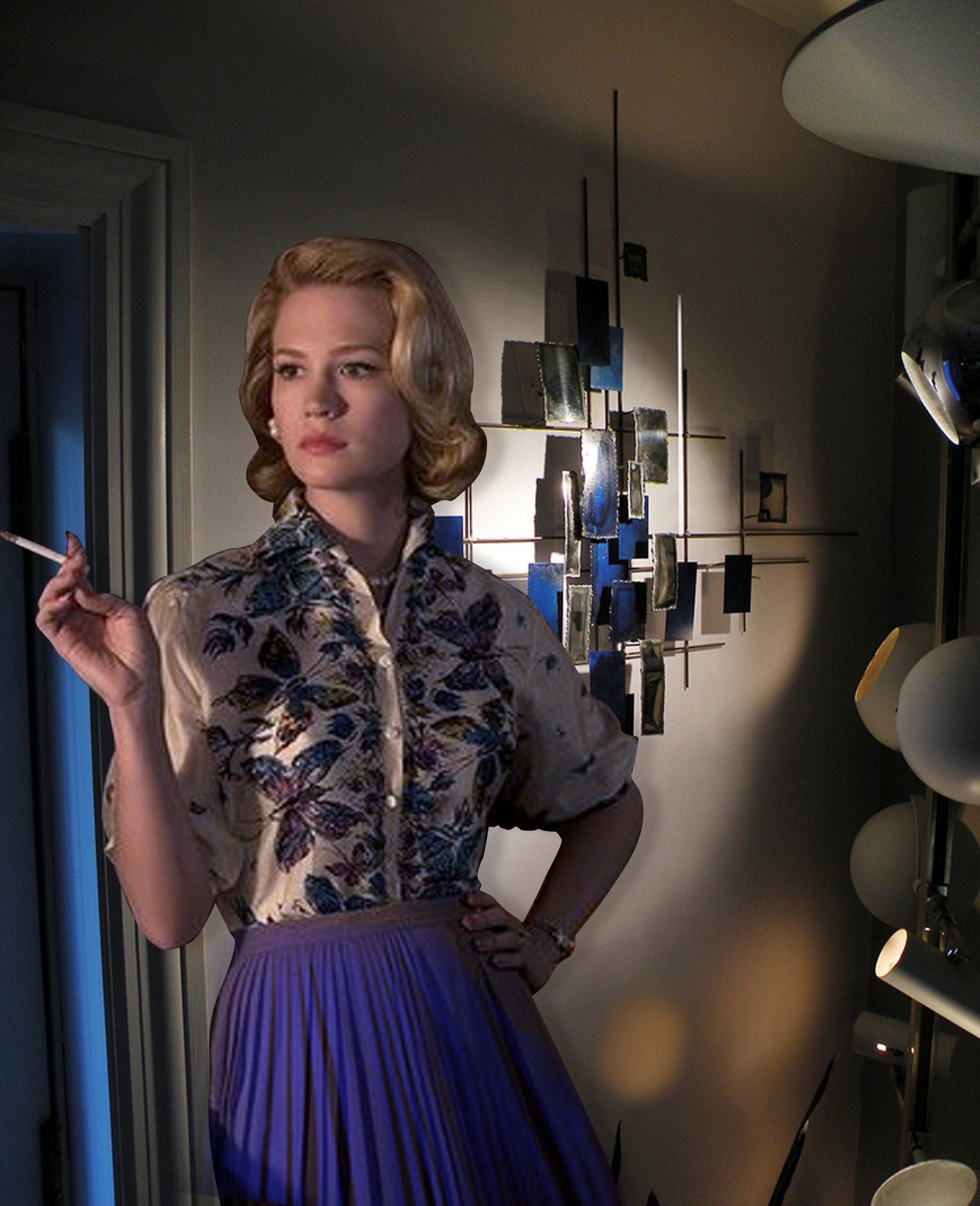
[[368, 571, 396, 585]]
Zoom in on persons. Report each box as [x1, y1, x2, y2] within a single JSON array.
[[35, 238, 649, 1206]]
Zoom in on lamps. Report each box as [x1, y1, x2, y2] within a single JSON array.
[[851, 279, 980, 1206]]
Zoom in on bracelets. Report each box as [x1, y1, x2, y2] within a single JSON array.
[[526, 913, 575, 962]]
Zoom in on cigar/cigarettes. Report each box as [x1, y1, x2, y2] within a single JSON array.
[[1, 531, 90, 575]]
